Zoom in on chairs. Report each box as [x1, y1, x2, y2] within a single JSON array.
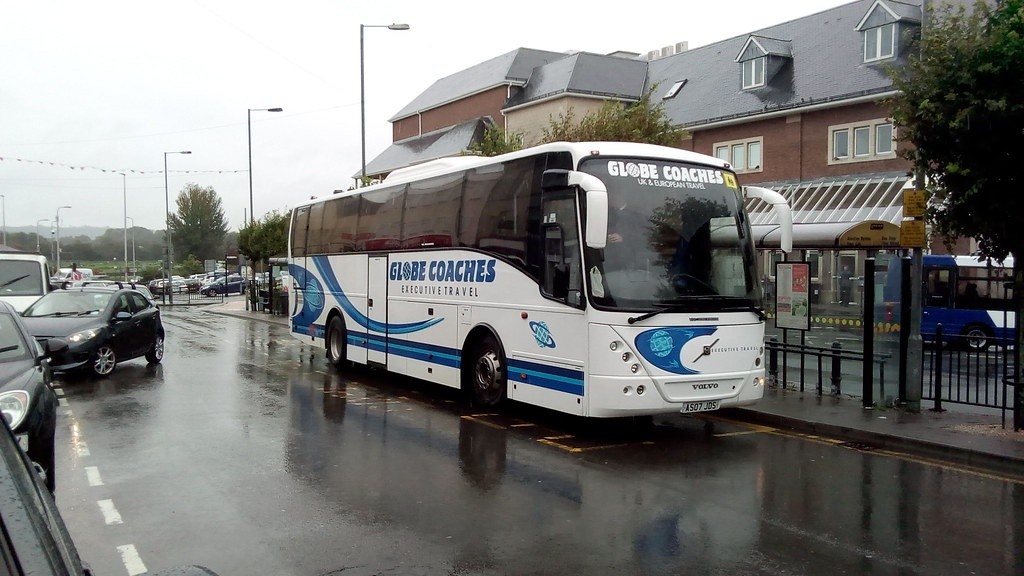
[[929, 281, 948, 299]]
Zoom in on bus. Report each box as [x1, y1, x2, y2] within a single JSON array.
[[288, 142, 792, 432]]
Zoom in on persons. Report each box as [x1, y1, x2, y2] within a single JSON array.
[[838, 266, 855, 307], [67, 263, 82, 280], [605, 188, 645, 272]]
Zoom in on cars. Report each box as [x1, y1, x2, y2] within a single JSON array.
[[203, 276, 249, 296], [0, 299, 56, 497], [1, 253, 50, 318], [151, 276, 202, 294], [22, 289, 165, 372]]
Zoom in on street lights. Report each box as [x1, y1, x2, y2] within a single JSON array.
[[164, 151, 193, 304], [248, 108, 283, 311], [360, 23, 409, 187], [120, 173, 137, 284], [1, 195, 7, 247], [37, 205, 71, 277]]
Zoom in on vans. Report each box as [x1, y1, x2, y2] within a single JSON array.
[[49, 268, 93, 290], [875, 251, 1021, 350]]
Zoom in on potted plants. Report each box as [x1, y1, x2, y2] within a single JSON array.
[[259, 287, 288, 315]]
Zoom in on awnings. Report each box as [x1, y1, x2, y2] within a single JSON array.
[[350, 116, 485, 178]]
